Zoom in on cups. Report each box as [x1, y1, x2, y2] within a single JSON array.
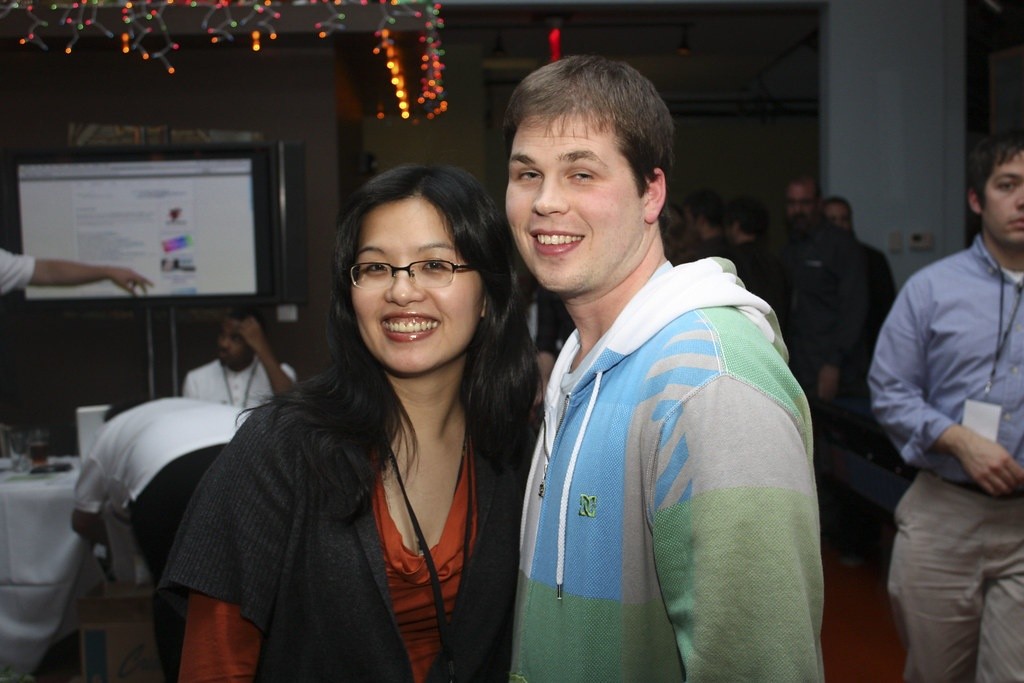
[[26, 431, 50, 465], [7, 430, 29, 474]]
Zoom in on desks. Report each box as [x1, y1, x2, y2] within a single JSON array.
[[0, 454, 152, 683]]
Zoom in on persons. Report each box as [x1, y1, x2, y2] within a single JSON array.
[[868, 129, 1023, 683], [660, 174, 897, 565], [0, 248, 153, 296], [158, 166, 540, 683], [504, 56, 826, 683], [530, 281, 576, 419], [71, 307, 296, 683]]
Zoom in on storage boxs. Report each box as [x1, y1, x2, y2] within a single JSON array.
[[75, 580, 167, 683]]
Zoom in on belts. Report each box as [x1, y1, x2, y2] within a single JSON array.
[[934, 475, 1024, 500]]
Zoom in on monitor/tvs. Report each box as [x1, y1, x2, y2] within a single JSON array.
[[15, 157, 258, 299]]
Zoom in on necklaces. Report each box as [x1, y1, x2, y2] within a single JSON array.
[[222, 365, 258, 408]]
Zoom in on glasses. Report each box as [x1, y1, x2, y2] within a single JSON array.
[[350, 259, 475, 289]]
